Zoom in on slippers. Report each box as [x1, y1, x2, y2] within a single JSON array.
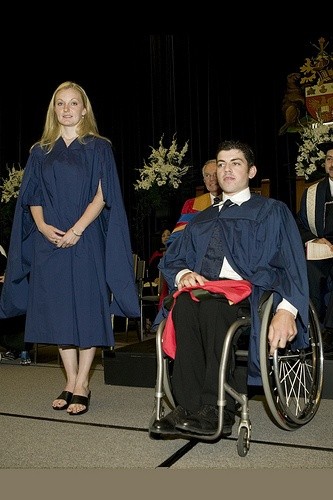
[[69, 391, 91, 415], [52, 391, 73, 410]]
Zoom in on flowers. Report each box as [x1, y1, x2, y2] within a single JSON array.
[[135, 132, 191, 190], [0, 163, 24, 203], [295, 126, 333, 181]]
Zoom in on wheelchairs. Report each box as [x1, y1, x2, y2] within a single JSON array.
[[149, 287, 325, 457]]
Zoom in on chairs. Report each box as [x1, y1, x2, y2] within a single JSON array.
[[110, 254, 161, 350]]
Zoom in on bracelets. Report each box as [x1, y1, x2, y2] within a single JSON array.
[[71, 228, 81, 236]]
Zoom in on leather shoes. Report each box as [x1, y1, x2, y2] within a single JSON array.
[[150, 405, 193, 433], [176, 405, 233, 433]]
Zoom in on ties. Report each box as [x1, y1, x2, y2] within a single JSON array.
[[199, 199, 234, 281], [214, 198, 220, 204]]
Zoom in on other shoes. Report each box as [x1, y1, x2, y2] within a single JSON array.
[[319, 328, 333, 357], [143, 322, 152, 334], [1, 352, 15, 360], [20, 355, 31, 365]]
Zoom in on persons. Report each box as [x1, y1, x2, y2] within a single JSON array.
[[149, 227, 172, 285], [298, 143, 333, 362], [169, 159, 217, 233], [152, 142, 298, 438], [0, 244, 31, 364], [29, 81, 110, 414]]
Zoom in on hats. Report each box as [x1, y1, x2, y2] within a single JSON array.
[[316, 142, 333, 155]]
